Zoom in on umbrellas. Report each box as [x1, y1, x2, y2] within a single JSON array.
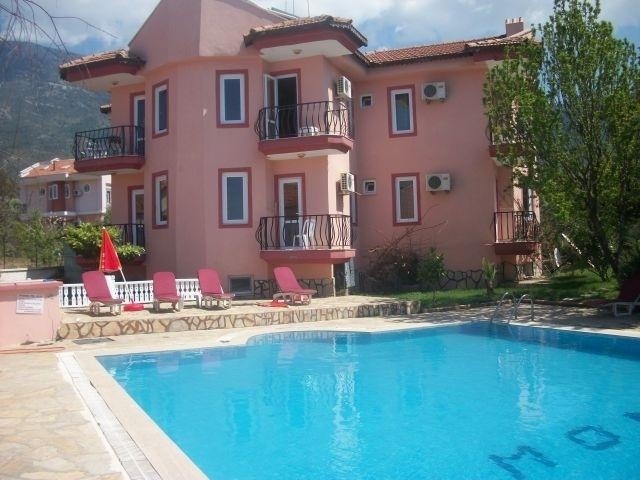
[[97, 225, 136, 303]]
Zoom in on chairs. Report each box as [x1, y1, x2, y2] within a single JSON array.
[[153, 271, 183, 312], [272, 266, 317, 306], [292, 219, 317, 249], [580, 279, 640, 317], [197, 268, 236, 310], [81, 270, 122, 317]]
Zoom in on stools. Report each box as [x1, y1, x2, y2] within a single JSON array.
[[298, 126, 319, 136]]
